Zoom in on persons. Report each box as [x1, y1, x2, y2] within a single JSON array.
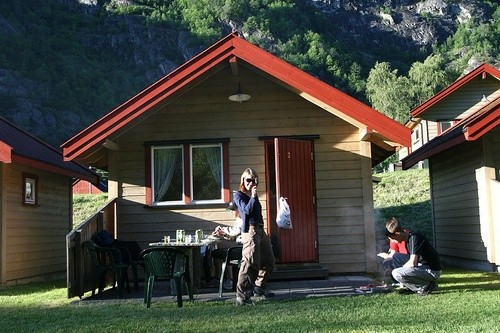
[[384, 216, 443, 295], [377, 228, 411, 284], [233, 168, 275, 305]]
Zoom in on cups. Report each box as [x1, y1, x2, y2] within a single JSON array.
[[176, 229, 204, 243], [164, 236, 170, 243]]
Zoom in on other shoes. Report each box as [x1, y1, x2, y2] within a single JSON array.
[[237, 296, 255, 306], [205, 278, 220, 288], [223, 279, 233, 289], [254, 291, 273, 298], [421, 282, 438, 295]]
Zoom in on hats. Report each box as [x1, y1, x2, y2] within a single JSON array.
[[226, 201, 237, 210]]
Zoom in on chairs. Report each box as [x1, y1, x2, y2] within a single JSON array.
[[81, 230, 243, 308]]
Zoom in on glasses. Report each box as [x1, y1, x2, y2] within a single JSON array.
[[245, 178, 255, 184]]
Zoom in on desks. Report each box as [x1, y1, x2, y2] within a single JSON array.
[[149, 237, 221, 295]]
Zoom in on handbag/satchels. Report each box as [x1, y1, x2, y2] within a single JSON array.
[[276, 197, 292, 229]]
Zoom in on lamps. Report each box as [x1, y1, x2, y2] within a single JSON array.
[[227, 82, 251, 105]]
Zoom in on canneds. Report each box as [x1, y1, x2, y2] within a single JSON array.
[[195, 228, 203, 243], [176, 229, 186, 242]]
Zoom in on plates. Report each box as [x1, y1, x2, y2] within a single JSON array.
[[162, 239, 176, 242]]
